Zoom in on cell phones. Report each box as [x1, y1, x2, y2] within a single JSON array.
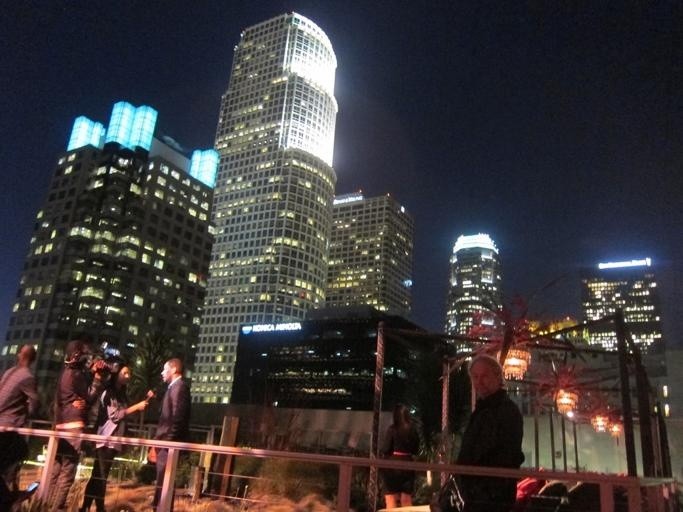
[[26, 481, 40, 494]]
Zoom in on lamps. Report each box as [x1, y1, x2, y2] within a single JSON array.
[[551, 386, 575, 421], [589, 414, 608, 432], [495, 348, 532, 384], [608, 421, 623, 437]]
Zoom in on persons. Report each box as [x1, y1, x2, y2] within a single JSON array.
[[47, 338, 108, 511], [0, 430, 34, 512], [78, 358, 152, 511], [0, 343, 40, 512], [435, 355, 524, 511], [150, 357, 191, 512], [375, 403, 420, 507]]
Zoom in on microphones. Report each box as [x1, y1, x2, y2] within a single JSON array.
[[145, 384, 161, 402]]
